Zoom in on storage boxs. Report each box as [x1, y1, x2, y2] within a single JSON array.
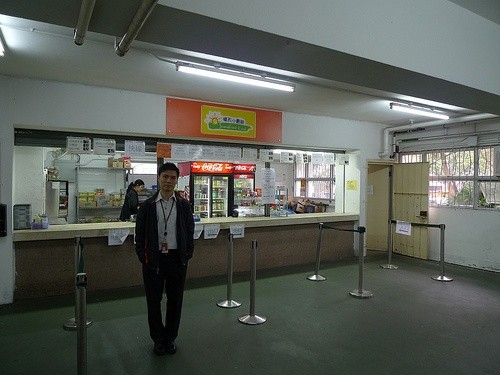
[[291, 202, 323, 214], [80, 192, 113, 207]]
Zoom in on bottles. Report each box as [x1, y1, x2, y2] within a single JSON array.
[[194, 177, 224, 219]]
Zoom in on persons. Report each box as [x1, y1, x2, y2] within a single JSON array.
[[119, 179, 145, 221], [135, 162, 195, 353]]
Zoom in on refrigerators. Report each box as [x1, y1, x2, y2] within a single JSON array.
[[177, 161, 233, 218], [233, 164, 256, 217]]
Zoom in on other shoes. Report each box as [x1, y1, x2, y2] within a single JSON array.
[[154, 339, 176, 355]]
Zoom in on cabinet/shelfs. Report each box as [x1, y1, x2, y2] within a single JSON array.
[[74, 167, 133, 224]]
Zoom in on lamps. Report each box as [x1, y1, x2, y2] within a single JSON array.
[[175, 60, 295, 93], [390, 100, 449, 121]]
